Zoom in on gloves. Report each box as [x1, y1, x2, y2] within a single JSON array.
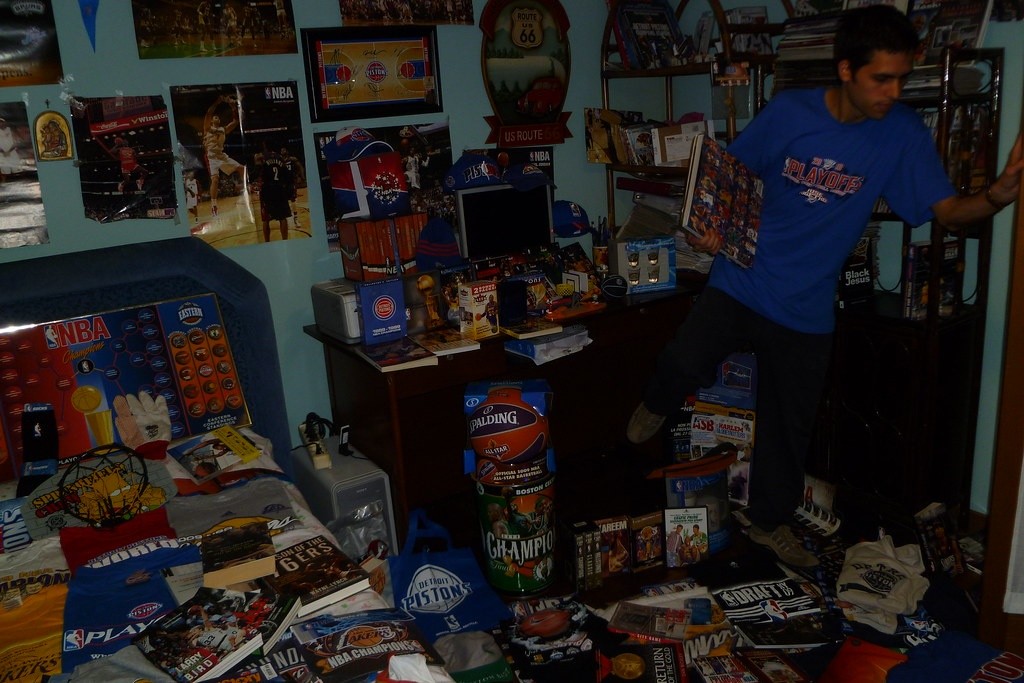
[[114, 391, 171, 460]]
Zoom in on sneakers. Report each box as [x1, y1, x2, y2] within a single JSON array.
[[749, 522, 819, 568], [626, 401, 666, 444]]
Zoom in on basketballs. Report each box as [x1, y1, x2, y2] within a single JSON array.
[[188, 605, 201, 615], [468, 388, 549, 465], [475, 313, 481, 321], [401, 139, 409, 148], [115, 138, 123, 145]]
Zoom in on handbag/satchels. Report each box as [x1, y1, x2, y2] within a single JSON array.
[[389, 508, 515, 644]]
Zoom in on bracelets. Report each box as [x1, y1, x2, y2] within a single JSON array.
[[983, 186, 1013, 210]]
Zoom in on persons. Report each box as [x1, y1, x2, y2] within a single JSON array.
[[667, 524, 685, 569], [478, 293, 499, 333], [931, 521, 963, 575], [522, 497, 553, 535], [148, 524, 363, 683], [688, 523, 708, 563], [0, 0, 475, 242], [624, 5, 1024, 528], [486, 503, 512, 564]]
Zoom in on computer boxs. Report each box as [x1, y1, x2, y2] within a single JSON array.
[[290, 434, 397, 565]]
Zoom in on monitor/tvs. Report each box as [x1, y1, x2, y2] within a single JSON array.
[[454, 182, 554, 263]]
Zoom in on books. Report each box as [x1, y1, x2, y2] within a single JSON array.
[[130, 595, 264, 683], [914, 501, 985, 578], [192, 521, 276, 588], [257, 536, 373, 617], [563, 506, 806, 683], [769, 0, 994, 321], [195, 584, 302, 656], [352, 279, 561, 372], [677, 134, 764, 269]]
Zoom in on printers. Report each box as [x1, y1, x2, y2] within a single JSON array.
[[312, 278, 362, 344]]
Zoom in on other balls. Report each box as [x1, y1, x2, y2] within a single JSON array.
[[601, 275, 627, 301]]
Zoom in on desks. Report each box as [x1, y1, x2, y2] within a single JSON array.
[[302, 268, 713, 556]]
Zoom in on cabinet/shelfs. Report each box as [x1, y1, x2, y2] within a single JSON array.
[[600, 0, 1003, 534]]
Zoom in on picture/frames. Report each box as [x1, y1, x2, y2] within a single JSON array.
[[300, 24, 444, 123]]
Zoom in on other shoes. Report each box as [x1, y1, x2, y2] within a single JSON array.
[[200, 48, 208, 52], [295, 219, 302, 227], [212, 206, 219, 216], [136, 180, 142, 190], [118, 184, 123, 192], [195, 217, 199, 224]]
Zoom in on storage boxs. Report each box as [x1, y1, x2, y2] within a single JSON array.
[[355, 280, 408, 346], [459, 278, 501, 341], [609, 234, 677, 294]]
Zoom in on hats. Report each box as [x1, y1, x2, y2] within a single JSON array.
[[323, 124, 393, 163]]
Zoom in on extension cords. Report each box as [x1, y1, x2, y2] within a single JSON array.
[[299, 422, 331, 470]]
